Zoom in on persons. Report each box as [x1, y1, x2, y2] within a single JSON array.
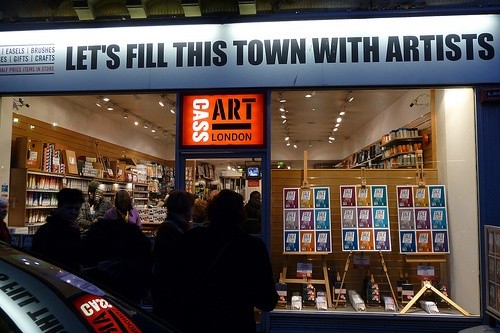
[[175, 189, 279, 333], [152, 191, 194, 319], [191, 197, 211, 227], [76, 180, 112, 230], [33, 187, 83, 270], [101, 189, 140, 227], [243, 191, 262, 220], [0, 200, 11, 244]]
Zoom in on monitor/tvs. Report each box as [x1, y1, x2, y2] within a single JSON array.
[[246, 166, 260, 179]]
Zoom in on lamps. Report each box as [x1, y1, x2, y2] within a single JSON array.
[[126, 0, 147, 19], [72, 0, 96, 20], [159, 93, 166, 107], [96, 95, 128, 120], [181, 0, 202, 17], [279, 90, 354, 148], [170, 102, 176, 114], [238, 0, 257, 15]]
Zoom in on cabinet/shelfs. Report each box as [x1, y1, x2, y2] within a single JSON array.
[[350, 137, 424, 168], [8, 169, 150, 226]]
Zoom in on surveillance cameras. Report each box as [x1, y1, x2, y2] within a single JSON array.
[[19, 98, 30, 108], [410, 97, 418, 107]]
